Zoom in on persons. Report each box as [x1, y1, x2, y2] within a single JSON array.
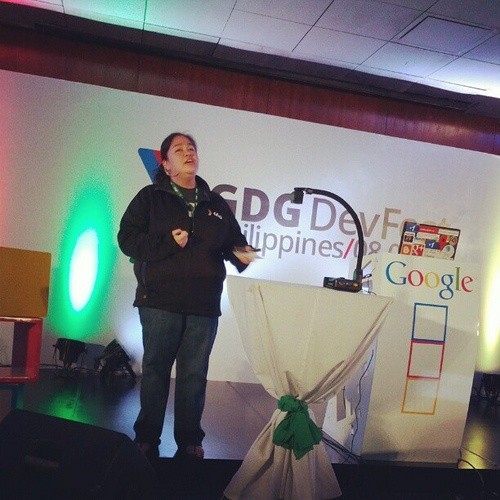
[[116, 133, 256, 457]]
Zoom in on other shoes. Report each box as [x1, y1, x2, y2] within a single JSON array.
[[176, 446, 204, 459], [135, 441, 161, 457]]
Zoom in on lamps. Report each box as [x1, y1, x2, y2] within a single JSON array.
[[53, 336, 86, 371], [94, 336, 137, 380]]
